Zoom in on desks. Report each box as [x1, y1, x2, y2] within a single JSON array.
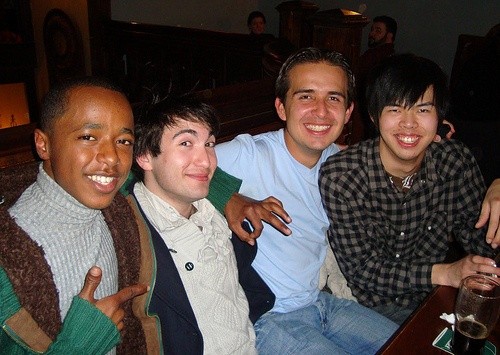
[[376, 249, 500, 354]]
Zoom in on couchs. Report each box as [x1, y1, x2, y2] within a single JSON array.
[[0, 20, 440, 355]]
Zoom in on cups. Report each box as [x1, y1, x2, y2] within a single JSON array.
[[450, 273, 500, 355]]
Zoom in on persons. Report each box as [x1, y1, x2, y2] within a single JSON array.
[[125, 94, 277, 355], [355, 15, 397, 141], [0, 75, 292, 355], [211, 48, 401, 355], [317, 52, 500, 326], [232, 11, 279, 70]]
